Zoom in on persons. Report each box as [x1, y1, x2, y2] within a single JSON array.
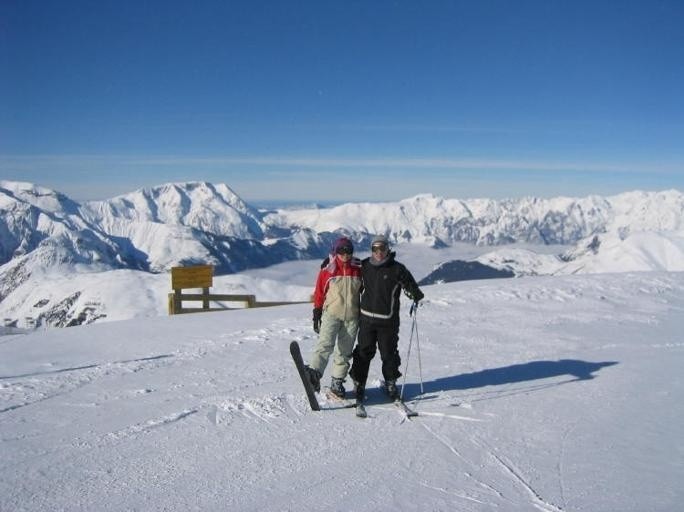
[[307, 238, 362, 397], [321, 236, 424, 402]]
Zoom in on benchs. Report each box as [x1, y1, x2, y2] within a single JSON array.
[[168, 292, 255, 315]]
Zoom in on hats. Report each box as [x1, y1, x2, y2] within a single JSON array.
[[371, 235, 389, 250], [334, 238, 353, 255]]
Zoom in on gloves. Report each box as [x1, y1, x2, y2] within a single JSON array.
[[313, 308, 322, 333], [413, 292, 424, 304]]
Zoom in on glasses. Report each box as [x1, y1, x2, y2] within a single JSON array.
[[336, 245, 353, 255], [372, 245, 387, 252]]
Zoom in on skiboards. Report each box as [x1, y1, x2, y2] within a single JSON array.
[[355, 381, 419, 418], [288, 341, 355, 411]]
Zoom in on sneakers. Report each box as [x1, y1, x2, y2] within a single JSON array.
[[330, 376, 345, 397], [354, 381, 365, 399], [383, 379, 398, 398], [303, 365, 322, 393]]
